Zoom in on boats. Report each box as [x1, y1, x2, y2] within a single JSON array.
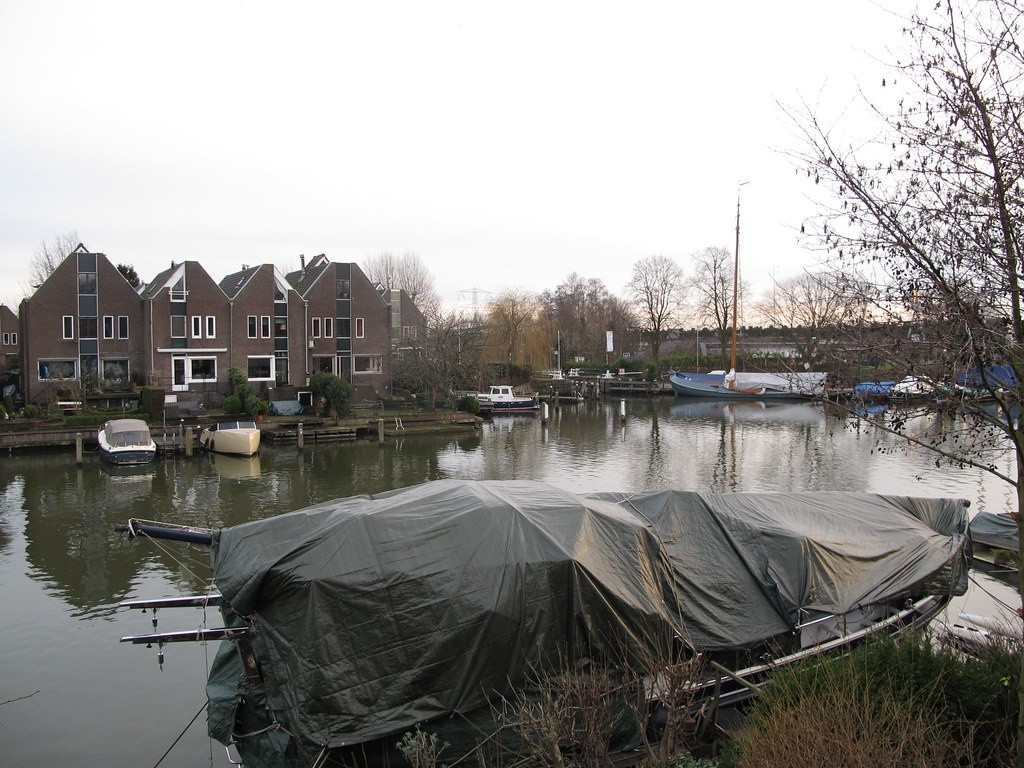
[[477, 398, 495, 414], [965, 509, 1020, 556], [849, 360, 1017, 406], [195, 418, 263, 460], [458, 384, 542, 412], [93, 413, 156, 466], [109, 481, 972, 750], [669, 181, 850, 400]]
